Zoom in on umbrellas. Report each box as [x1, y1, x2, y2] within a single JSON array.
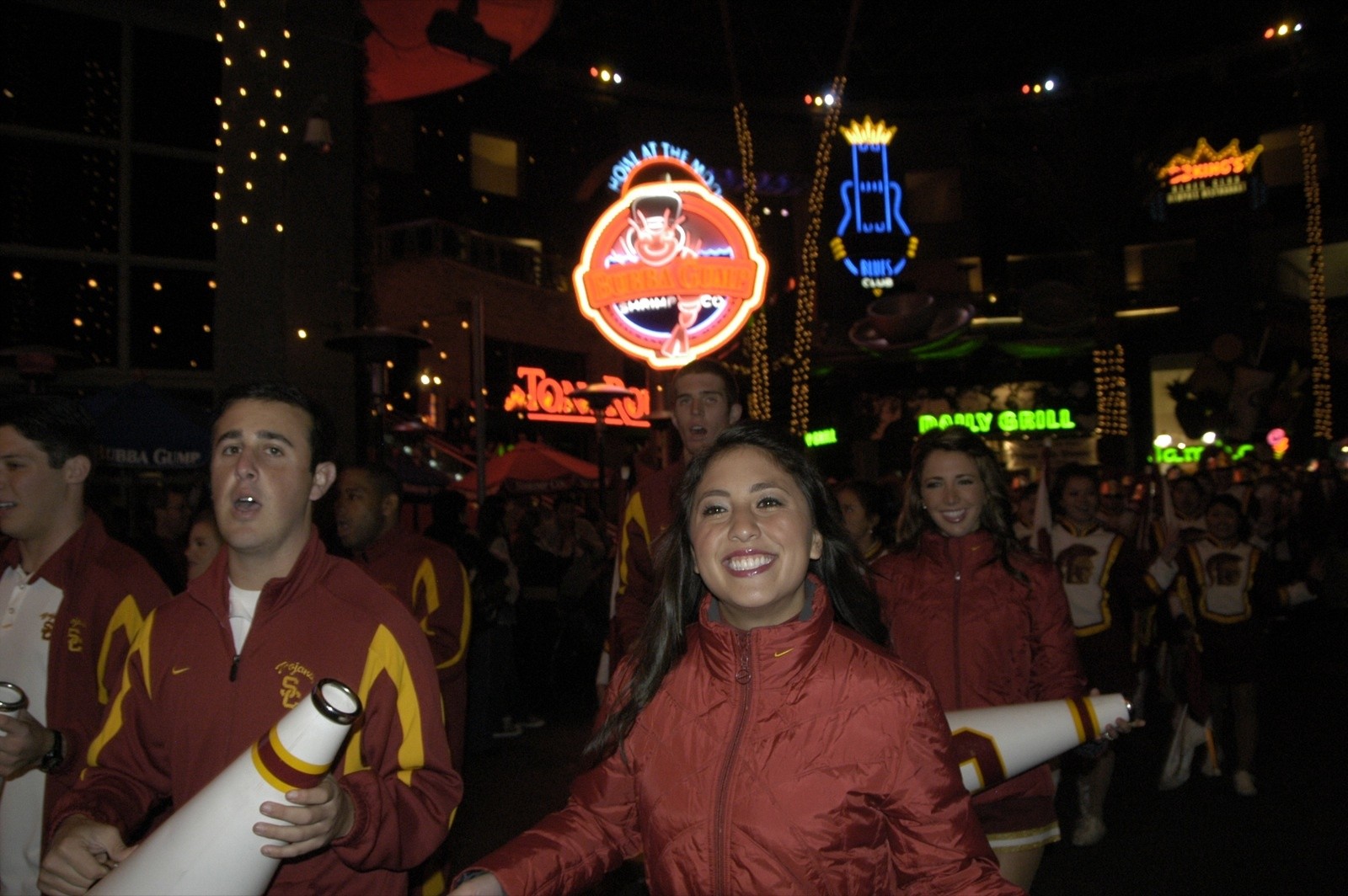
[[444, 433, 600, 493]]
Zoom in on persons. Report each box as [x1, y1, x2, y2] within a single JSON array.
[[0, 383, 175, 896], [597, 359, 747, 720], [36, 376, 464, 896], [442, 420, 1018, 896], [862, 427, 1146, 896], [0, 427, 1348, 896]]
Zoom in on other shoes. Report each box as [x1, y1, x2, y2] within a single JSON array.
[[1233, 770, 1256, 799], [1200, 759, 1220, 775]]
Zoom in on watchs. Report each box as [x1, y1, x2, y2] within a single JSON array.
[[37, 729, 63, 775]]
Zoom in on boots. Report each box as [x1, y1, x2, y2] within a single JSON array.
[[1074, 749, 1111, 846], [1159, 703, 1205, 790]]
